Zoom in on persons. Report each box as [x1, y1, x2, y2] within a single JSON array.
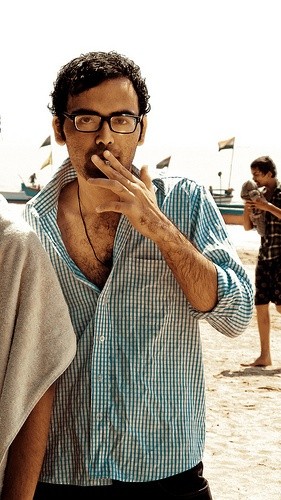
[[21, 50, 254, 500], [28, 173, 37, 187], [240, 156, 281, 367], [0, 191, 76, 500]]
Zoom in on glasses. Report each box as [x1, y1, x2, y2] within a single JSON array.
[[63, 112, 144, 135]]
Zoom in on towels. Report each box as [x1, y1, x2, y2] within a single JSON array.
[[0, 193, 77, 486]]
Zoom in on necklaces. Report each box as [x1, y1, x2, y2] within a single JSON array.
[[77, 185, 114, 268]]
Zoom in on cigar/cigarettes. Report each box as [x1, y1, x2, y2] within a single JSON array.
[[103, 159, 110, 165]]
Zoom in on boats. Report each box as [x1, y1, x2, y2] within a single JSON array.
[[0, 135, 246, 225]]
